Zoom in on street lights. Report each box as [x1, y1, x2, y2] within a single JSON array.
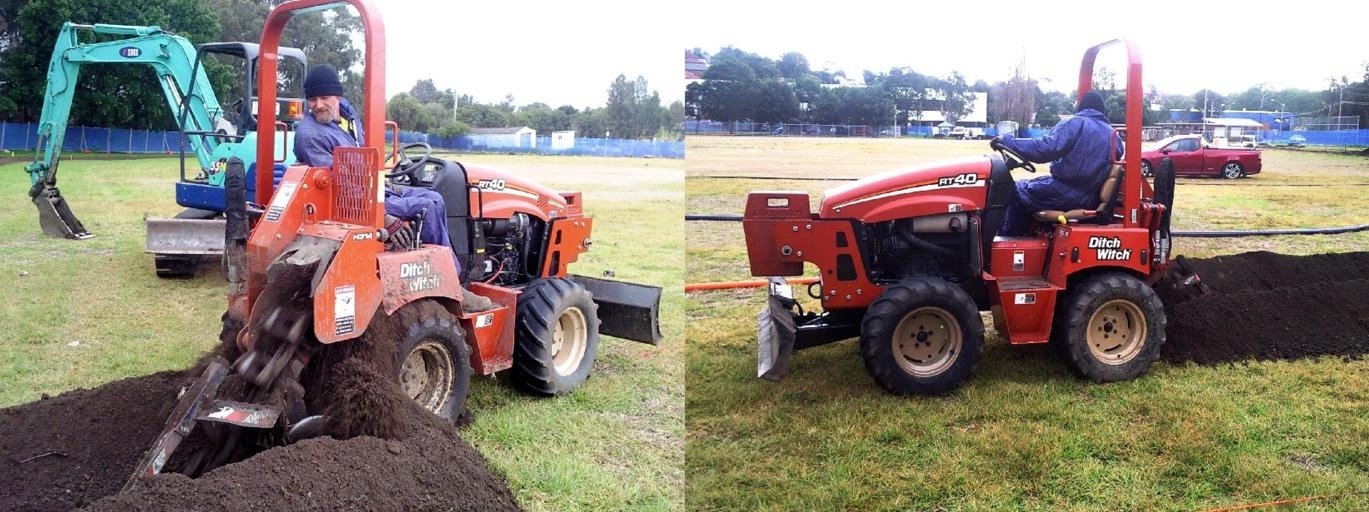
[[1271, 99, 1285, 139]]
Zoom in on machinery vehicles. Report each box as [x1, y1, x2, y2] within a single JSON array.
[[23, 18, 307, 279], [124, 2, 664, 495], [742, 39, 1206, 398]]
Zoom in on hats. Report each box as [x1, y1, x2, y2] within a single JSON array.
[[304, 64, 343, 99], [1079, 92, 1104, 111]]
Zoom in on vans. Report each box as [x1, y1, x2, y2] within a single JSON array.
[[1217, 135, 1258, 148]]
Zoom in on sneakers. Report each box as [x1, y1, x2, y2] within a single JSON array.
[[461, 287, 491, 312]]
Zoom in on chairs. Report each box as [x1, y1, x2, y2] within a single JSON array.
[[1032, 160, 1124, 225]]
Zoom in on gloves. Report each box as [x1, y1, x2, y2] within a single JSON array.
[[383, 213, 415, 248], [990, 136, 1003, 151]]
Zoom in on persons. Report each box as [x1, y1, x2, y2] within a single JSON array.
[[291, 67, 491, 315], [989, 90, 1125, 235]]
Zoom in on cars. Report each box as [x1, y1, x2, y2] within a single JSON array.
[[1289, 135, 1306, 143]]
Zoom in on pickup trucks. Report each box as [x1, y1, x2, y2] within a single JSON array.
[[1123, 134, 1263, 179]]
